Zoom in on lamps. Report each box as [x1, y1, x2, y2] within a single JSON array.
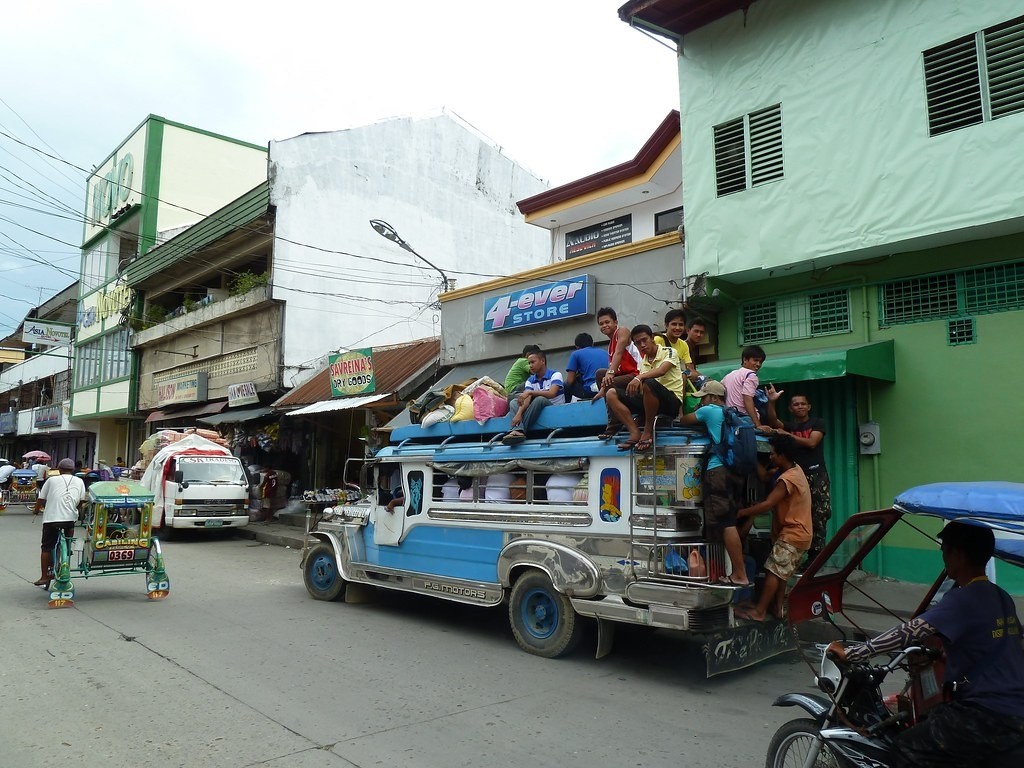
[[370, 218, 448, 292]]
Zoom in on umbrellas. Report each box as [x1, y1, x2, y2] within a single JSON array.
[[22, 450, 51, 460]]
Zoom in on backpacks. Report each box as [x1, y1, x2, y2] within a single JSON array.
[[694, 402, 757, 476]]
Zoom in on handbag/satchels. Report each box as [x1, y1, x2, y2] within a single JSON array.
[[682, 378, 701, 416], [742, 372, 768, 406]]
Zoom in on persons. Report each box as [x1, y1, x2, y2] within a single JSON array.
[[562, 333, 608, 402], [591, 306, 712, 454], [826, 521, 1024, 768], [765, 382, 831, 577], [0, 456, 125, 489], [733, 433, 812, 622], [502, 349, 565, 446], [33, 458, 85, 586], [680, 380, 758, 586], [504, 344, 540, 393], [719, 345, 773, 433]]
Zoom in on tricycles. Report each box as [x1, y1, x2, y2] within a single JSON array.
[[42, 471, 171, 608], [0, 469, 41, 513]]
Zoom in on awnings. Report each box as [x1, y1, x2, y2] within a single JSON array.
[[145, 401, 229, 423], [696, 338, 896, 386]]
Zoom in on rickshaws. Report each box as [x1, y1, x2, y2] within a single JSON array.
[[764, 478, 1024, 768]]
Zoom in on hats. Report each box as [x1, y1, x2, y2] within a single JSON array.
[[57, 458, 75, 469], [691, 379, 726, 398]]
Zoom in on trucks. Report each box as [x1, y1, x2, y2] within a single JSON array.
[[118, 453, 254, 537]]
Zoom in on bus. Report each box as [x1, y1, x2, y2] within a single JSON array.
[[298, 398, 801, 678]]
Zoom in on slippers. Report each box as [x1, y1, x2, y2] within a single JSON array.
[[718, 576, 749, 588], [618, 432, 657, 452], [34, 580, 47, 585], [734, 609, 769, 624], [501, 432, 526, 445]]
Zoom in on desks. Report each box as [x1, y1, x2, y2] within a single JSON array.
[[300, 501, 338, 534]]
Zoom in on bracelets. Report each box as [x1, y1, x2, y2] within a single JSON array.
[[634, 377, 640, 381], [35, 509, 39, 513], [608, 370, 614, 374]]
